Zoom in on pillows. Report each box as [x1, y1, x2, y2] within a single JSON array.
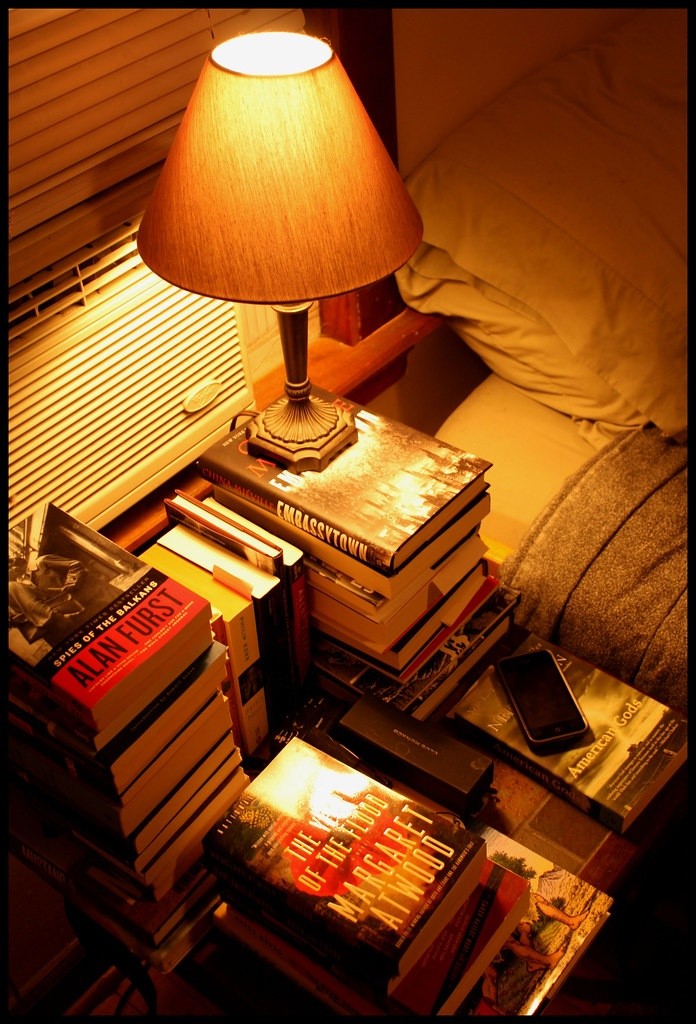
[[395, 8, 687, 444]]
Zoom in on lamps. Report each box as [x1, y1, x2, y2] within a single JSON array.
[[135, 30, 427, 476]]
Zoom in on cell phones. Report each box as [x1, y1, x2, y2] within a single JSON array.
[[496, 649, 588, 747]]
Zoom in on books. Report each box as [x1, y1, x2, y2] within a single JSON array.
[[455, 633, 688, 833], [6, 380, 615, 1016]]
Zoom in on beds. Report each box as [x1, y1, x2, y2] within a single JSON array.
[[303, 8, 687, 915]]
